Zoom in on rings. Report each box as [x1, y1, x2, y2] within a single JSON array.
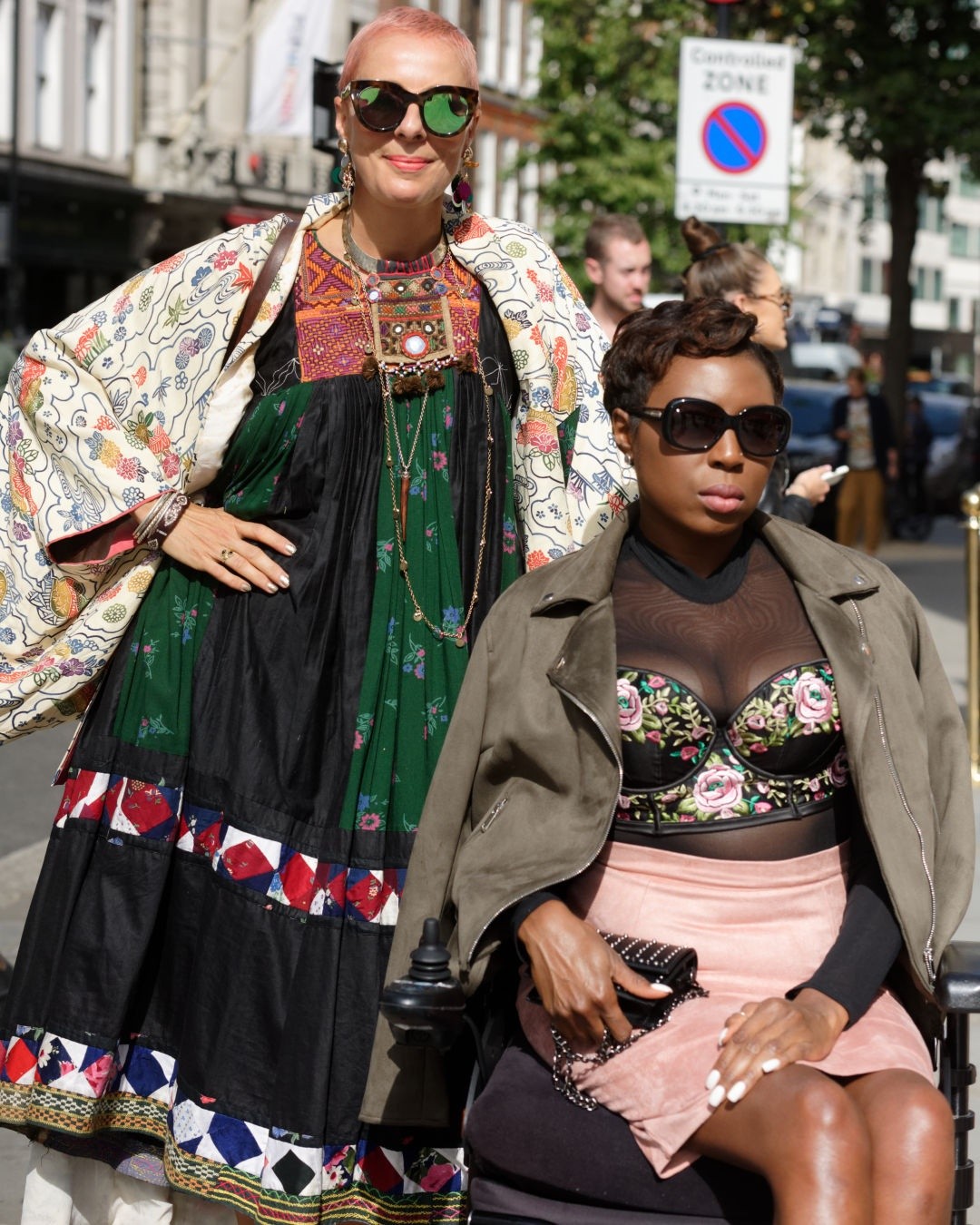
[[221, 548, 234, 559]]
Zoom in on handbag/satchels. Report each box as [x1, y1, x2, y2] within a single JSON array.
[[525, 929, 711, 1113]]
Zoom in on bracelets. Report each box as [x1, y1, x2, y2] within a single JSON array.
[[132, 491, 190, 551]]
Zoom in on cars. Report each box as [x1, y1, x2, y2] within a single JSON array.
[[780, 378, 851, 487], [918, 390, 980, 513]]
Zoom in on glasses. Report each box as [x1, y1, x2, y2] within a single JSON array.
[[339, 80, 480, 138], [747, 290, 794, 319], [624, 396, 793, 459]]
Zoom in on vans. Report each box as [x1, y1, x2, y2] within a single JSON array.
[[790, 340, 864, 383]]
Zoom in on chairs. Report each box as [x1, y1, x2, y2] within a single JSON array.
[[377, 918, 979, 1224]]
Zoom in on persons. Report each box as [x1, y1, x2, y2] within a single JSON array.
[[584, 211, 653, 347], [679, 215, 849, 526], [831, 365, 899, 555], [904, 398, 934, 545], [0, 0, 641, 1225], [357, 291, 975, 1225]]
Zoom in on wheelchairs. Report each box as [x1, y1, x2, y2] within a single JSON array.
[[377, 916, 980, 1225]]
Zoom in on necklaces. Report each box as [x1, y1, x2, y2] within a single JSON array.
[[341, 197, 493, 641]]
